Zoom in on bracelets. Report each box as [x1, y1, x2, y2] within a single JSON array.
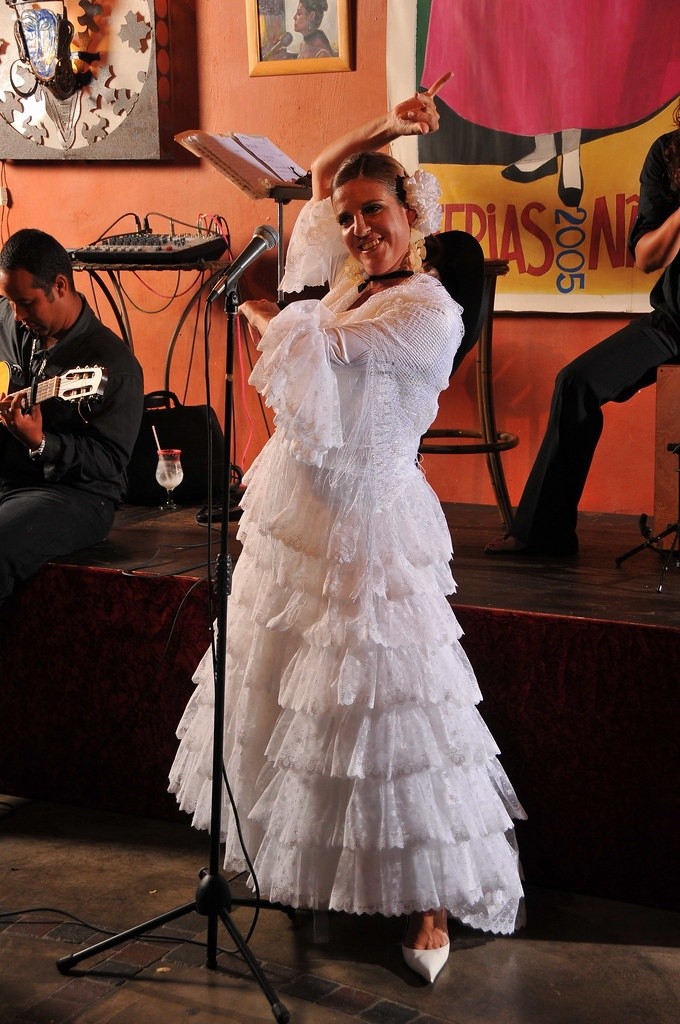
[[29, 435, 46, 461]]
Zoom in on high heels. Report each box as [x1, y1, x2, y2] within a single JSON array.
[[401, 912, 451, 985]]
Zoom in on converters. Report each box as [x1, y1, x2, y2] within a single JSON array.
[[0, 186, 8, 206]]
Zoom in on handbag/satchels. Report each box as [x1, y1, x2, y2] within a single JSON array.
[[126, 389, 223, 504]]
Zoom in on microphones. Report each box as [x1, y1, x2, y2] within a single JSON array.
[[206, 224, 279, 304], [261, 32, 293, 61]]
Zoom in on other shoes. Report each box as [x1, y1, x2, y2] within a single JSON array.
[[484, 526, 580, 555]]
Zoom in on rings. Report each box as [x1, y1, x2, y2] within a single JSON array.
[[8, 422, 13, 428]]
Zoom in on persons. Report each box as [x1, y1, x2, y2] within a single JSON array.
[[293, 0, 332, 59], [0, 229, 144, 601], [166, 71, 525, 984], [484, 100, 680, 566]]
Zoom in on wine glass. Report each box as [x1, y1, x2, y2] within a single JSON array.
[[155, 449, 184, 510]]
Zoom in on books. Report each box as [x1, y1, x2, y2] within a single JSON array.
[[183, 132, 311, 197]]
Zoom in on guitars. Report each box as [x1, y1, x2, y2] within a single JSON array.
[[0, 360, 111, 422]]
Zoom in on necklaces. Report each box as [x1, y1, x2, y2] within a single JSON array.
[[358, 270, 413, 293]]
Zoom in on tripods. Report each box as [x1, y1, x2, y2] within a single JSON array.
[[56, 295, 302, 1021]]
